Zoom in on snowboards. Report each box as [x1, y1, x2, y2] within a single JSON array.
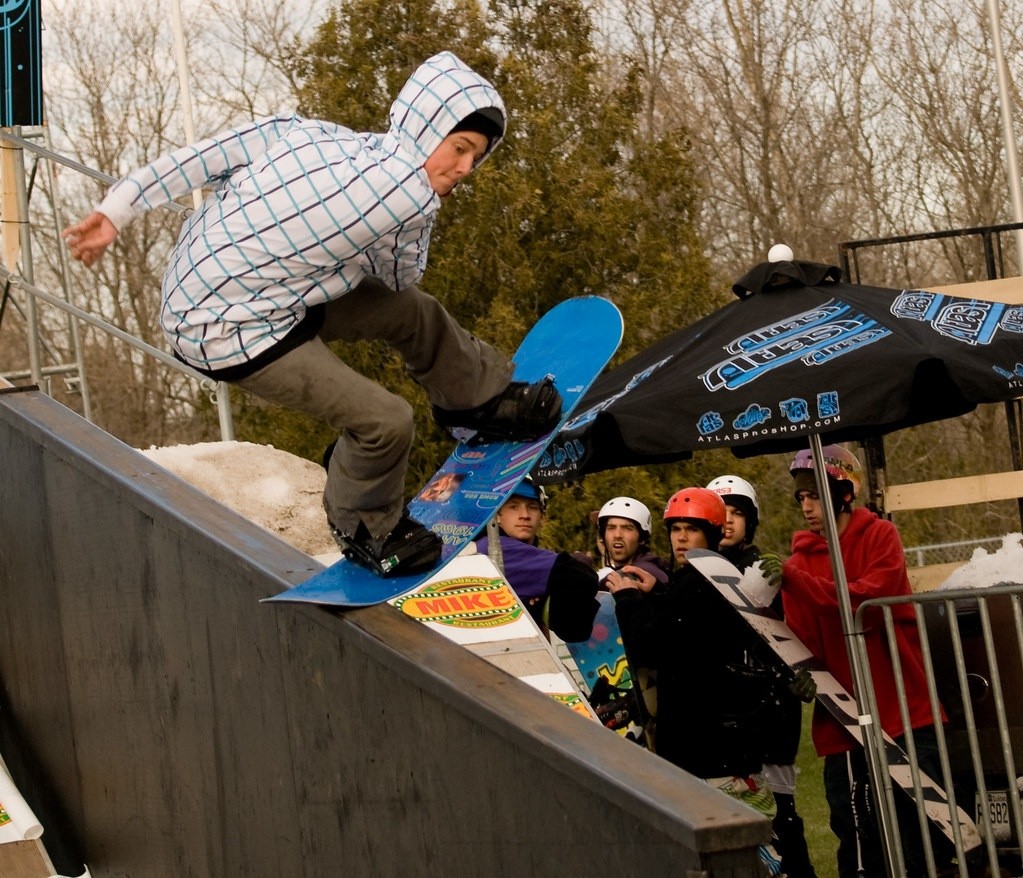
[[560, 588, 647, 749], [681, 545, 987, 866], [254, 293, 626, 611]]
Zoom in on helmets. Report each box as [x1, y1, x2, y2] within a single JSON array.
[[511, 473, 549, 512], [705, 475, 762, 527], [597, 497, 653, 544], [662, 486, 727, 541], [790, 443, 864, 505]]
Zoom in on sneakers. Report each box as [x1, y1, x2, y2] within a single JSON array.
[[326, 506, 443, 578], [432, 374, 563, 448]]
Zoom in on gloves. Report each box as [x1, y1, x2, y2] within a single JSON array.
[[789, 666, 818, 703], [758, 547, 784, 586]]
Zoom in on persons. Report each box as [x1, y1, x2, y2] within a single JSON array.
[[475, 482, 599, 700], [566, 495, 666, 751], [705, 473, 819, 878], [606, 486, 785, 878], [760, 445, 950, 878], [61, 49, 565, 579]]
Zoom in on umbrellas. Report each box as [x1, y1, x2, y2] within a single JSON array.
[[530, 243, 1023, 878]]
